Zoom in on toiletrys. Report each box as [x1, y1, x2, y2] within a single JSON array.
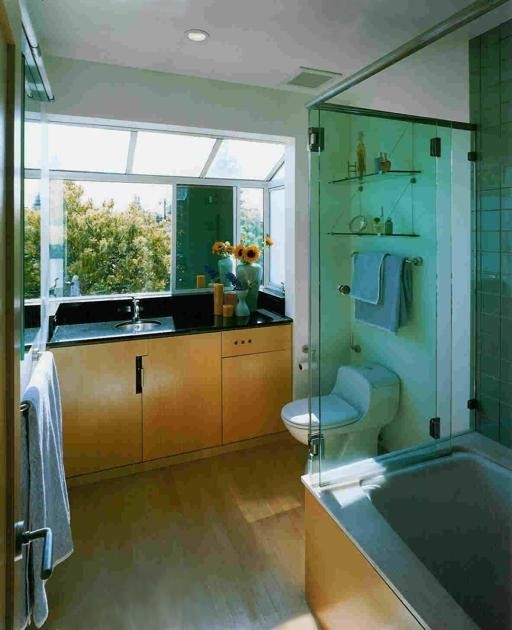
[[356, 132, 392, 234]]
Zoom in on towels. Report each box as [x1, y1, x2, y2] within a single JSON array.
[[17, 348, 75, 630], [347, 250, 416, 336]]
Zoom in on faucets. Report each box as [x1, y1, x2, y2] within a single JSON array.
[[130, 296, 143, 324]]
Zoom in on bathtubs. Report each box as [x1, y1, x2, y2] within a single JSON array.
[[300, 431, 512, 630]]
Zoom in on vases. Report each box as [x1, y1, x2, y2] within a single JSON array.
[[216, 258, 234, 287], [208, 283, 217, 288], [237, 261, 265, 315], [232, 286, 251, 321]]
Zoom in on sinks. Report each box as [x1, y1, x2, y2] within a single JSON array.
[[115, 320, 161, 330]]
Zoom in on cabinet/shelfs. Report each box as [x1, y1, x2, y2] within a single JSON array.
[[325, 168, 426, 238], [38, 321, 222, 490], [217, 319, 295, 455]]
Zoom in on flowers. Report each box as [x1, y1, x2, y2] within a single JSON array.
[[204, 264, 220, 286], [224, 271, 244, 291], [230, 234, 278, 266], [209, 236, 234, 262]]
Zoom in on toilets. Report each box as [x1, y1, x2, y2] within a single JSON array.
[[281, 362, 400, 473]]
